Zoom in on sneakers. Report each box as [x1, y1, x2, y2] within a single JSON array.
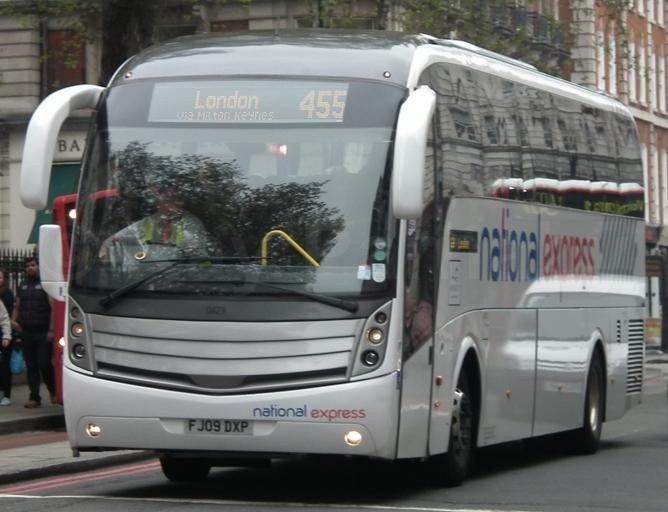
[[0, 397, 11, 405], [23, 394, 57, 408]]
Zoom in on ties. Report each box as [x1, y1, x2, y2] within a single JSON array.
[[160, 218, 174, 244]]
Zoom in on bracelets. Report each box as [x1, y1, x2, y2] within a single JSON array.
[[48, 327, 55, 332]]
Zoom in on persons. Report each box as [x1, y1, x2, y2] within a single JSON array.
[[99, 178, 215, 263], [0, 300, 13, 348], [11, 256, 61, 406], [0, 268, 14, 406]]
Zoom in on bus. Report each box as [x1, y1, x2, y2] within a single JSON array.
[[45, 183, 159, 406], [16, 27, 654, 490], [491, 176, 646, 220]]
